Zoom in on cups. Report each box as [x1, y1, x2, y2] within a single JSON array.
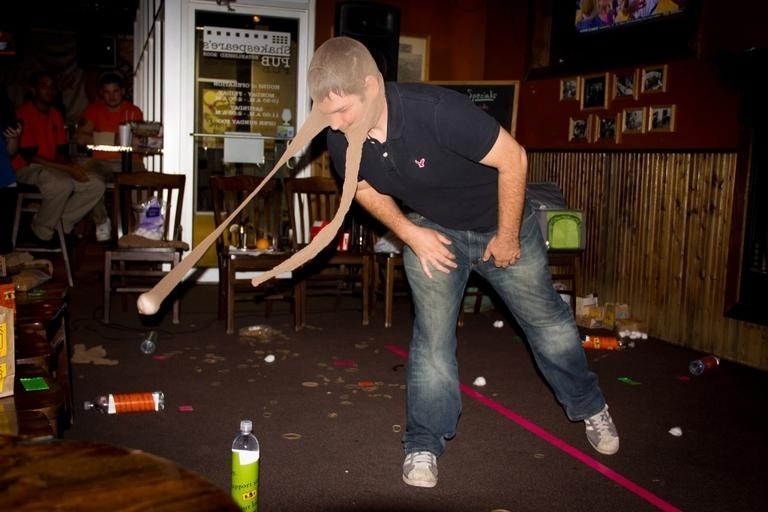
[[118, 125, 131, 147], [353, 223, 366, 253]]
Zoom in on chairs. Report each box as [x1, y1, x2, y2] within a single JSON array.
[[15, 351, 52, 377], [13, 410, 55, 438], [103, 172, 185, 325], [16, 374, 70, 439]]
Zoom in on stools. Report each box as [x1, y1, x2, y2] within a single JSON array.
[[102, 185, 136, 238], [10, 184, 76, 288]]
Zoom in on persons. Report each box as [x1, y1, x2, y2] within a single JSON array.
[[0, 70, 143, 259], [306, 37, 620, 488]]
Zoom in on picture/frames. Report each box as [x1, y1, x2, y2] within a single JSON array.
[[558, 64, 675, 145], [399, 32, 431, 84], [425, 80, 521, 140], [723, 121, 768, 327]]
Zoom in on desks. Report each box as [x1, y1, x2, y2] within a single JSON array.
[[87, 144, 160, 236], [0, 433, 245, 511]]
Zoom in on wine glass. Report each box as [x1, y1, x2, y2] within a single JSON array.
[[281, 109, 292, 126]]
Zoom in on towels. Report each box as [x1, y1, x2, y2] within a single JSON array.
[[222, 131, 267, 166]]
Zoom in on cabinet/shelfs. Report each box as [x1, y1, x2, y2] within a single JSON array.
[[14, 300, 75, 424]]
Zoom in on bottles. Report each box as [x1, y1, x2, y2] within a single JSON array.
[[231, 420, 260, 512], [690, 355, 720, 376], [85, 391, 165, 415], [582, 334, 635, 351]]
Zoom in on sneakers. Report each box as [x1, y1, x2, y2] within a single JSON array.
[[584, 404, 620, 455], [402, 449, 439, 488], [95, 217, 111, 242]]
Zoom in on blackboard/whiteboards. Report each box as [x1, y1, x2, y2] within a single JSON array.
[[427, 79, 518, 134]]
